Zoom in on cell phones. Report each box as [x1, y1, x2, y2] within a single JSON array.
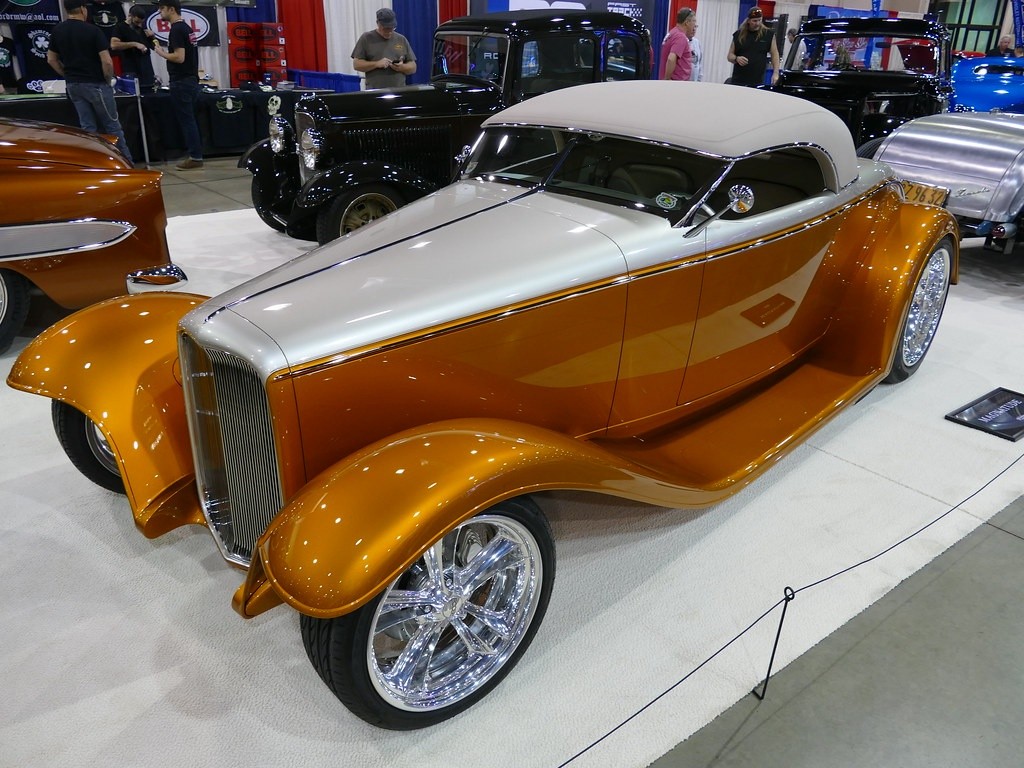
[[390, 59, 401, 69]]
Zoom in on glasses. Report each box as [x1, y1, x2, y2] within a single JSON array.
[[749, 10, 762, 18], [683, 9, 692, 21], [383, 26, 396, 33]]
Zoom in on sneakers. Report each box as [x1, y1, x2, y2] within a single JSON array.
[[174, 158, 205, 170]]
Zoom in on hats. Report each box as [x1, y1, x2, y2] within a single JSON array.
[[64, 0, 88, 8], [151, 0, 180, 6], [376, 8, 397, 28]]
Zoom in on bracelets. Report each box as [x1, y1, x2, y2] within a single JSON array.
[[735, 56, 738, 62]]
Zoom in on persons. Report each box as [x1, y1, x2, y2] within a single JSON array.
[[985, 35, 1014, 57], [687, 23, 704, 82], [47, 0, 136, 167], [727, 7, 780, 85], [1014, 46, 1024, 58], [151, 0, 206, 171], [659, 7, 696, 81], [351, 8, 418, 90], [787, 29, 807, 71], [111, 5, 155, 88], [0, 35, 23, 95], [804, 38, 818, 71]]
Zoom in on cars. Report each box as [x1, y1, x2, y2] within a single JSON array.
[[6, 80, 960, 732], [0, 117, 189, 355], [855, 50, 1024, 254], [238, 8, 653, 245], [754, 17, 954, 149]]
